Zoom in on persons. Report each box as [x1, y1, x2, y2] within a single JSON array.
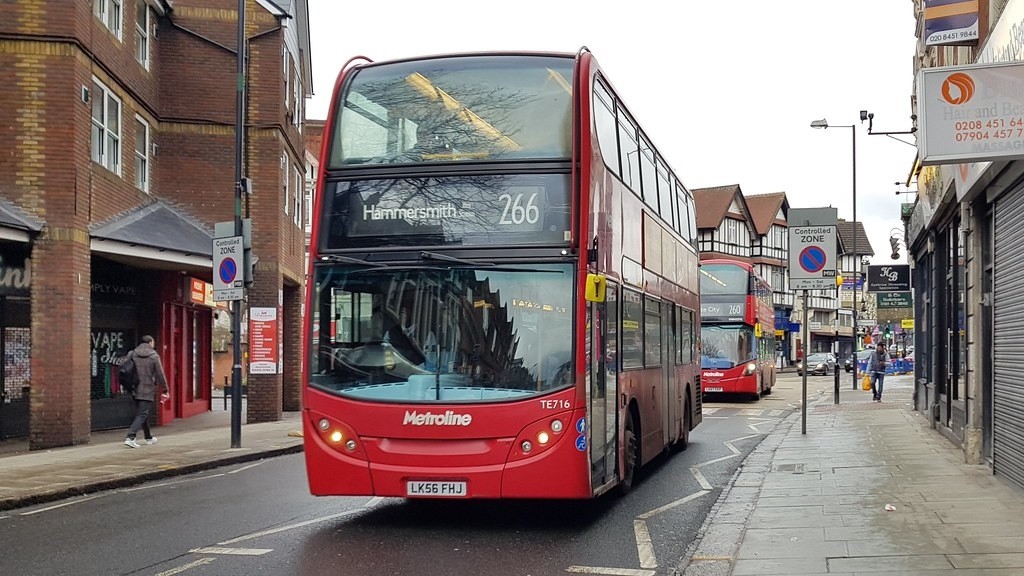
[[866, 343, 891, 402], [123, 335, 169, 448]]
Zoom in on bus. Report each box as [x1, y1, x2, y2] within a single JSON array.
[[298, 42, 704, 503], [697, 258, 778, 403]]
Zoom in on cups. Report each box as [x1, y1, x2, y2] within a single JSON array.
[[160, 394, 167, 404]]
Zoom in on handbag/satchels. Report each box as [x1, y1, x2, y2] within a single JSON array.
[[862, 373, 872, 391]]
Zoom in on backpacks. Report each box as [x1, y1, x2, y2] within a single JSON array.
[[118, 353, 139, 395]]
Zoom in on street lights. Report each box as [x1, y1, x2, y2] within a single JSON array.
[[809, 119, 858, 391]]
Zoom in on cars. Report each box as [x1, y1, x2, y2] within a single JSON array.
[[797, 352, 838, 376], [845, 349, 877, 373], [889, 343, 914, 370]]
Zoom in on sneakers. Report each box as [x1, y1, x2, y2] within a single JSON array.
[[872, 394, 882, 403], [124, 437, 141, 449], [144, 437, 157, 445]]
